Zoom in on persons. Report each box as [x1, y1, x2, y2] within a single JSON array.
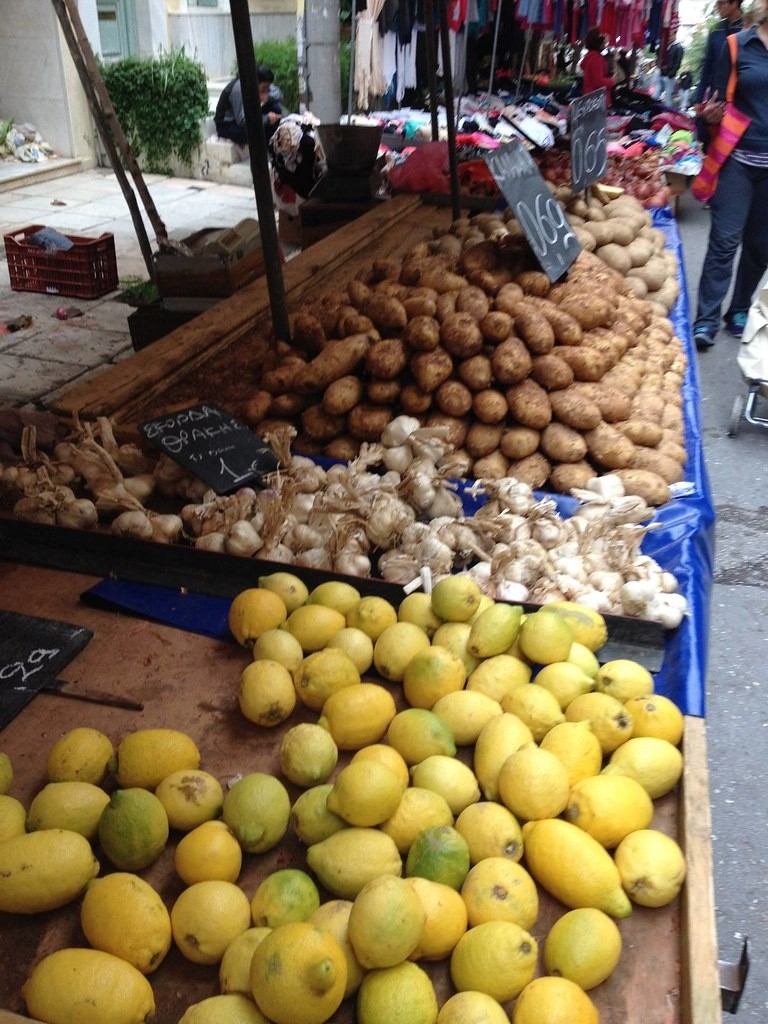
[[214, 65, 281, 157], [579, 27, 684, 110], [692, 1, 768, 350], [696, 0, 745, 209]]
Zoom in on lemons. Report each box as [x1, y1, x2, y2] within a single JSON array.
[[0, 573, 688, 1024]]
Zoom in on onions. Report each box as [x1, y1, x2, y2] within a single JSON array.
[[460, 148, 671, 208]]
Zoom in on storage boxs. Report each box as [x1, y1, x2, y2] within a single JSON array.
[[2, 224, 119, 301], [127, 196, 391, 354]]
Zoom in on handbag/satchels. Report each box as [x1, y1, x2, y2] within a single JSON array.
[[690, 34, 752, 201]]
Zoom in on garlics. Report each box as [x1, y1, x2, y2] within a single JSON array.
[[0, 417, 690, 628]]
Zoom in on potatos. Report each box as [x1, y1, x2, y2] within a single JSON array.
[[241, 185, 689, 508]]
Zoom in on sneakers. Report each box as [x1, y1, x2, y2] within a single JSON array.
[[725, 312, 746, 337], [693, 326, 716, 345]]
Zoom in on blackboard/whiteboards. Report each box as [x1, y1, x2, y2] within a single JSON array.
[[0, 610, 94, 732], [139, 402, 284, 495], [568, 86, 607, 195], [483, 137, 583, 284]]
